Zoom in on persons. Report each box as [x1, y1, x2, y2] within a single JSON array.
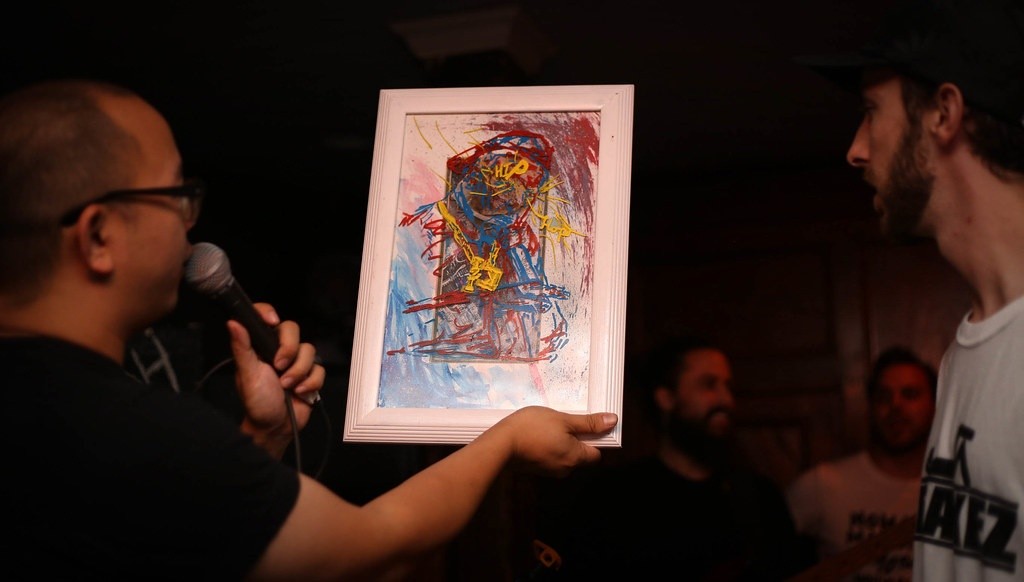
[[584, 333, 817, 582], [787, 4, 1023, 582], [0, 81, 618, 580], [784, 342, 934, 582]]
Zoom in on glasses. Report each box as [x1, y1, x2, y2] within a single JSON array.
[[57, 186, 201, 234]]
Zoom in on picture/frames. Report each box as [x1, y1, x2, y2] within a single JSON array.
[[340, 83, 634, 450]]
